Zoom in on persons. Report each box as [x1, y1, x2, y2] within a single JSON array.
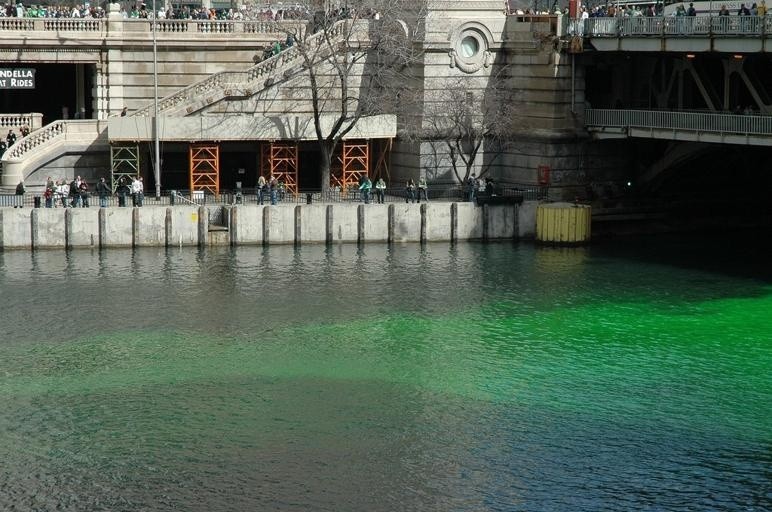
[[121, 106, 128, 117], [510, 0, 769, 36], [0, 0, 380, 63], [467, 172, 494, 202], [405, 177, 416, 203], [359, 174, 386, 204], [417, 176, 428, 203], [255, 173, 285, 205], [14, 179, 25, 208], [42, 175, 145, 207], [0, 124, 30, 159]]
